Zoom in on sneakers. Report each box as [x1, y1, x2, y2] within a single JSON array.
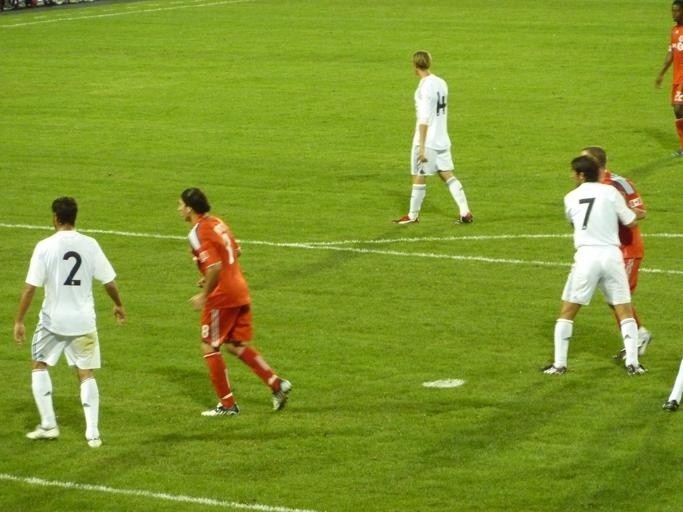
[[200, 401, 239, 417], [25, 424, 60, 440], [662, 400, 679, 411], [539, 364, 567, 375], [271, 379, 294, 411], [455, 213, 473, 224], [87, 438, 102, 448], [613, 330, 652, 375], [391, 216, 420, 224]]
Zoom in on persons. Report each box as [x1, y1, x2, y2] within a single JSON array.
[[661, 359, 683, 412], [654, 0, 683, 159], [391, 51, 474, 226], [539, 156, 648, 377], [176, 187, 294, 418], [580, 147, 651, 362], [13, 196, 126, 448]]
